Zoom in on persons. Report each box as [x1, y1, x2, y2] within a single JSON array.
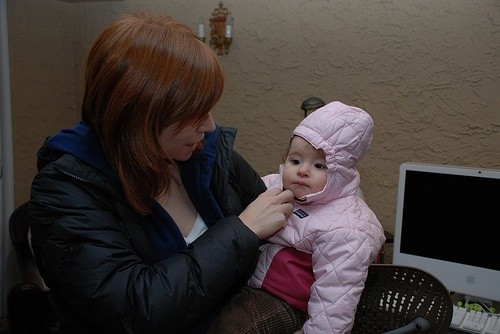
[[27, 11, 294, 334], [206, 100, 386, 334]]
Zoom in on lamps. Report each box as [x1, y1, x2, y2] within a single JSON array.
[[198, 0, 235, 57]]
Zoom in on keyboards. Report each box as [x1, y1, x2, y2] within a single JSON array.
[[381, 289, 499, 334]]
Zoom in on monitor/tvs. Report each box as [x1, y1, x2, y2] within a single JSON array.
[[392, 162, 500, 302]]
